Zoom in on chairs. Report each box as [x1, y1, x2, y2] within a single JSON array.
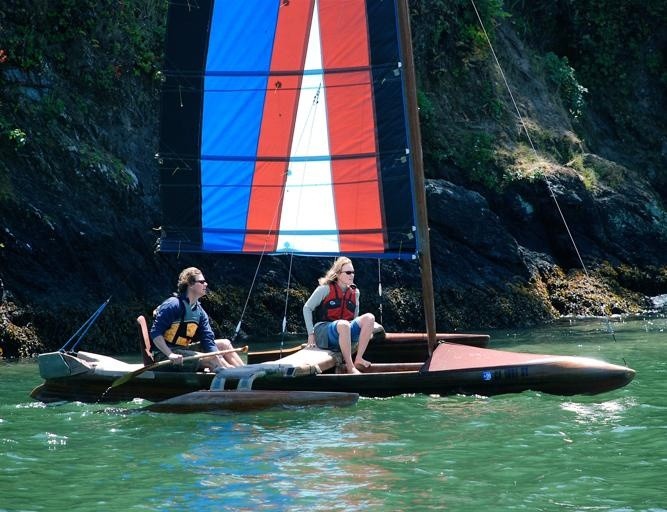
[[136, 315, 157, 370]]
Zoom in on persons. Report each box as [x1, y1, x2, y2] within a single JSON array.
[[302, 256, 377, 373], [150, 267, 243, 369]]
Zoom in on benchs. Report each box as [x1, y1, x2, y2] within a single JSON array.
[[258, 343, 359, 380]]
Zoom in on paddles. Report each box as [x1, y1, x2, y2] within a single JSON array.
[[112, 346, 249, 387]]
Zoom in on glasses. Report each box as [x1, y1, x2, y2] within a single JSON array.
[[340, 271, 356, 274], [194, 280, 208, 284]]
